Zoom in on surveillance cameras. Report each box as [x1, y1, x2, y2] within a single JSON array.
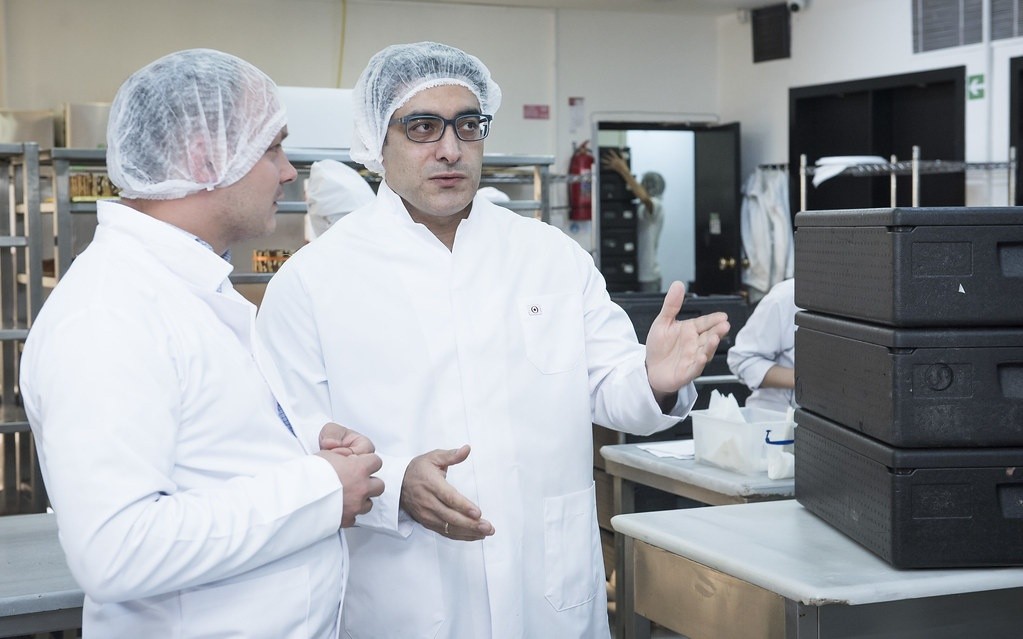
[[786, 0, 806, 13]]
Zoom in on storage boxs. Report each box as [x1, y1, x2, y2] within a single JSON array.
[[56, 102, 108, 149], [278, 86, 365, 149], [0, 110, 54, 150], [599, 146, 640, 291], [794, 207, 1023, 571]]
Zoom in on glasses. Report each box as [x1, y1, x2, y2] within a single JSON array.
[[387, 114, 492, 143]]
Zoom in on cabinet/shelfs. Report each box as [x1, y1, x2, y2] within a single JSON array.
[[0, 140, 554, 515]]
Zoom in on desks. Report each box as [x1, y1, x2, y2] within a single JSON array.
[[599, 439, 794, 514], [609, 500, 1023, 639], [0, 513, 84, 639]]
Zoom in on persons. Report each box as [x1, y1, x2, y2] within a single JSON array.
[[256, 41, 730, 639], [19, 50, 384, 639], [601, 149, 665, 293], [727, 278, 801, 415]]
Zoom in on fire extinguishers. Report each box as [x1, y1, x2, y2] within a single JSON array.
[[568, 140, 596, 220]]
